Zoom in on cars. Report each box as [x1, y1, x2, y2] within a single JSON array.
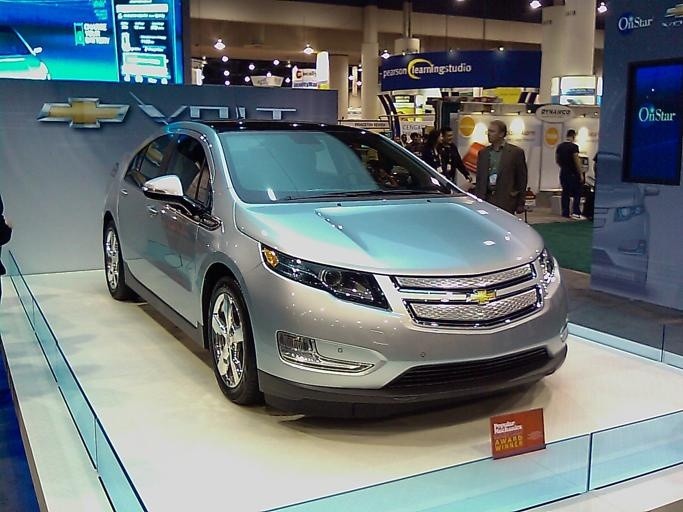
[[100, 104, 572, 425], [0, 24, 51, 82], [591, 150, 661, 293]]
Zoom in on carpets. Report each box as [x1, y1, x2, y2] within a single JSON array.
[[527, 218, 590, 277]]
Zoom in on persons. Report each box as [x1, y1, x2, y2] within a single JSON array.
[[387, 133, 426, 162], [437, 125, 472, 193], [473, 119, 528, 217], [0, 192, 12, 305], [554, 129, 586, 220], [422, 128, 444, 176]]
